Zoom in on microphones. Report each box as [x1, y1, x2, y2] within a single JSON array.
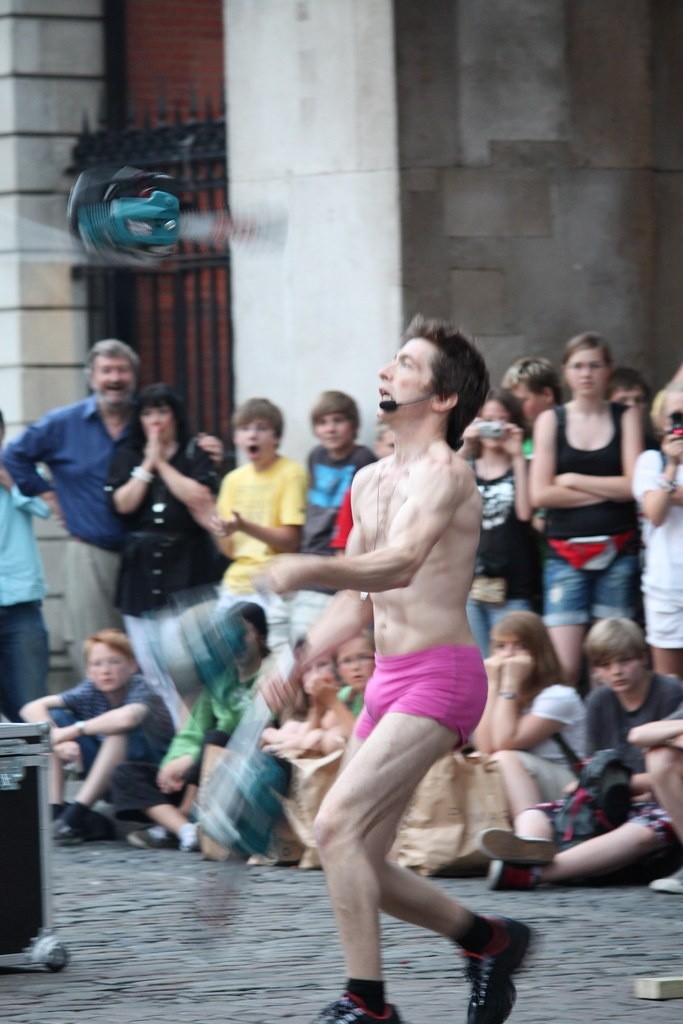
[[379, 393, 436, 410]]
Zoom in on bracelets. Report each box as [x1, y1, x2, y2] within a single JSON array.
[[496, 691, 522, 700], [130, 466, 153, 485]]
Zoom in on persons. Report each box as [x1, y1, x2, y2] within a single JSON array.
[[266, 313, 532, 1024], [0, 332, 683, 894]]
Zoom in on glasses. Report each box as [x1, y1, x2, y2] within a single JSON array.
[[336, 653, 374, 666], [564, 361, 609, 371]]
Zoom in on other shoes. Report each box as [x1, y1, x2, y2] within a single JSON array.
[[126, 828, 180, 849]]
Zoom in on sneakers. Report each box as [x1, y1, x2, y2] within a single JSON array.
[[310, 992, 401, 1024], [648, 868, 683, 894], [52, 819, 82, 846], [487, 860, 541, 890], [477, 828, 556, 864], [455, 914, 531, 1024]]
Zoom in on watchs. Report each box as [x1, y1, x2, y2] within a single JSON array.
[[75, 721, 85, 736]]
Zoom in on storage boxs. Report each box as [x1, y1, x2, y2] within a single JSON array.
[[0, 722, 71, 975]]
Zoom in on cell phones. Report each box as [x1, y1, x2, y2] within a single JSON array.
[[673, 413, 683, 439]]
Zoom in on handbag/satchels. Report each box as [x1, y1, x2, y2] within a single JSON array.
[[545, 528, 642, 571], [470, 575, 508, 605], [553, 752, 635, 845]]
